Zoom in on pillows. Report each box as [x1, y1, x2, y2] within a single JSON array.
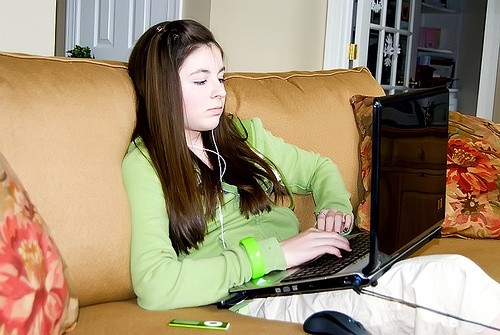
[[0, 153, 78, 335], [349, 94, 500, 238]]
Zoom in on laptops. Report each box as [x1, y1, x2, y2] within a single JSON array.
[[229, 87, 449, 299]]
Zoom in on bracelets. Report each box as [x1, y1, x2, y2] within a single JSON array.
[[239, 237, 266, 279]]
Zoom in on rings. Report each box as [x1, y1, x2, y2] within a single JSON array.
[[318, 212, 326, 216]]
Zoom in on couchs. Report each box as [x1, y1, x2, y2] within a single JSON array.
[[1, 52, 500, 335]]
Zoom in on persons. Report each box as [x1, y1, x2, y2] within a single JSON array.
[[121, 19, 499, 334]]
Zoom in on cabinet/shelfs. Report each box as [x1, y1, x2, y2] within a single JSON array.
[[417, 0, 463, 89]]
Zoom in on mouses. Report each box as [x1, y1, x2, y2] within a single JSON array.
[[303, 311, 371, 334]]
